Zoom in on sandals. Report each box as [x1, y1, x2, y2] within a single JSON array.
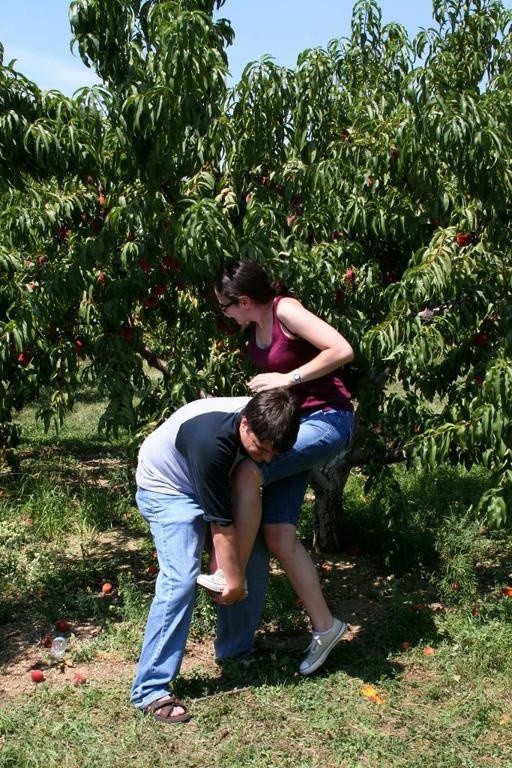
[[143, 693, 190, 723]]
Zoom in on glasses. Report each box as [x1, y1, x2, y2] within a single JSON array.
[[218, 301, 237, 311]]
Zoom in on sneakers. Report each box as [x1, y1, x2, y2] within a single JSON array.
[[299, 618, 346, 675], [196, 568, 248, 597]]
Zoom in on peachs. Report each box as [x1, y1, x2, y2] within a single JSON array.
[[246, 129, 487, 387], [32, 669, 44, 683], [149, 565, 157, 575], [503, 586, 512, 597], [102, 582, 113, 594], [57, 619, 68, 632], [73, 672, 87, 687], [16, 176, 187, 368]]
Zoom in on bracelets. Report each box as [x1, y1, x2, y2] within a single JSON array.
[[292, 368, 302, 384]]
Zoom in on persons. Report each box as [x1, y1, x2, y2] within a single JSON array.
[[127, 388, 301, 725], [192, 259, 357, 675]]
